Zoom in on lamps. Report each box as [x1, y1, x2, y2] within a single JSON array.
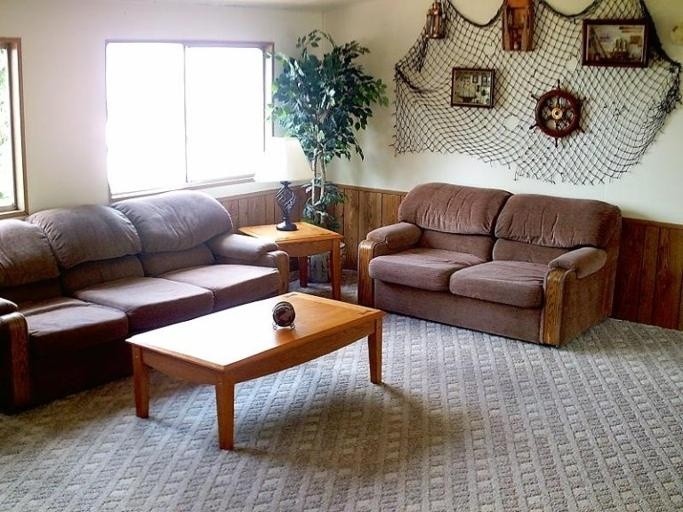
[[252, 135, 315, 232]]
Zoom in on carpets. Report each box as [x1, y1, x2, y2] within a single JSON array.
[[1, 316, 683, 511]]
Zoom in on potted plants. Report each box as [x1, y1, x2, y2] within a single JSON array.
[[262, 28, 391, 284]]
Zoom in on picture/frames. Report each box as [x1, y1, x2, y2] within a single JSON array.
[[449, 66, 495, 109], [581, 17, 650, 68]]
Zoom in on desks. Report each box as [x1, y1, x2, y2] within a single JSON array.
[[237, 220, 345, 301]]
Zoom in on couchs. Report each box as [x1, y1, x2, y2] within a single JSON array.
[[356, 180, 625, 351], [0, 188, 291, 415]]
